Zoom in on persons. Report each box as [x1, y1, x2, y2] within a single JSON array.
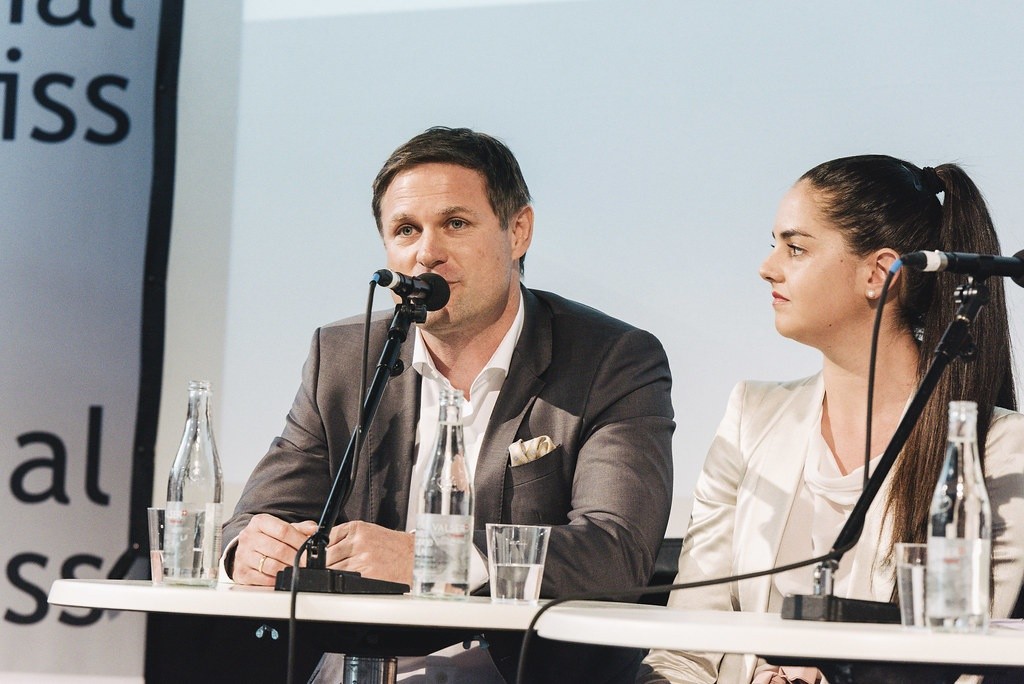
[[633, 155, 1024, 684], [217, 125, 675, 683]]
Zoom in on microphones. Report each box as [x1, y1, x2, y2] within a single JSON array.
[[907, 248, 1024, 288], [374, 269, 450, 311]]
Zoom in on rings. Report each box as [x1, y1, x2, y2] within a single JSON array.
[[258, 556, 267, 573]]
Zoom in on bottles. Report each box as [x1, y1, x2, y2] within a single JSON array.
[[167, 379, 225, 587], [927, 400, 993, 630], [411, 387, 474, 596]]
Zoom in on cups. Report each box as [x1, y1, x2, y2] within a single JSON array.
[[146, 508, 205, 587], [895, 542, 929, 626], [485, 522, 552, 603]]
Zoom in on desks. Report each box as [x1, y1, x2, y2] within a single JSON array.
[[47, 579, 1024, 667]]
